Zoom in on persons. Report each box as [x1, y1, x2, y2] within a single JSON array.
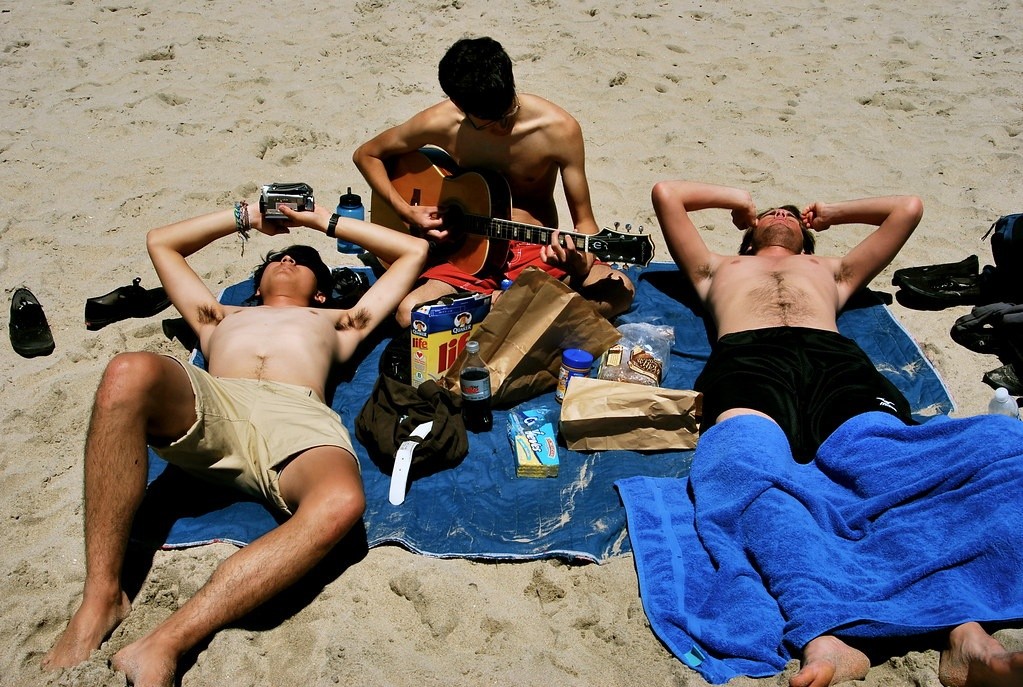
[[39, 201, 430, 687], [340, 37, 636, 329], [651, 178, 1023, 687]]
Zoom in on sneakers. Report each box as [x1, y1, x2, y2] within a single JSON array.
[[10, 289, 55, 358], [86, 276, 172, 330]]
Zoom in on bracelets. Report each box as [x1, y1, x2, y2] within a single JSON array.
[[326, 213, 341, 237], [234, 200, 251, 241]]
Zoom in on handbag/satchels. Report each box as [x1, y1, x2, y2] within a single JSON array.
[[359, 376, 468, 479], [951, 214, 1023, 360]]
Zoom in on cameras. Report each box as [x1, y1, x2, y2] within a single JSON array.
[[259, 183, 315, 219]]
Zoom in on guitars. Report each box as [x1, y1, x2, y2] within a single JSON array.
[[368, 146, 656, 292]]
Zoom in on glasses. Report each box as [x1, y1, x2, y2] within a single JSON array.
[[467, 88, 522, 131]]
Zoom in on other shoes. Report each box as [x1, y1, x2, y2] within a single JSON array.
[[982, 360, 1023, 396], [895, 256, 979, 300]]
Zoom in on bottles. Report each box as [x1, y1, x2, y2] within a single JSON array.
[[987, 388, 1020, 423], [460, 341, 493, 434], [336, 186, 367, 254]]
